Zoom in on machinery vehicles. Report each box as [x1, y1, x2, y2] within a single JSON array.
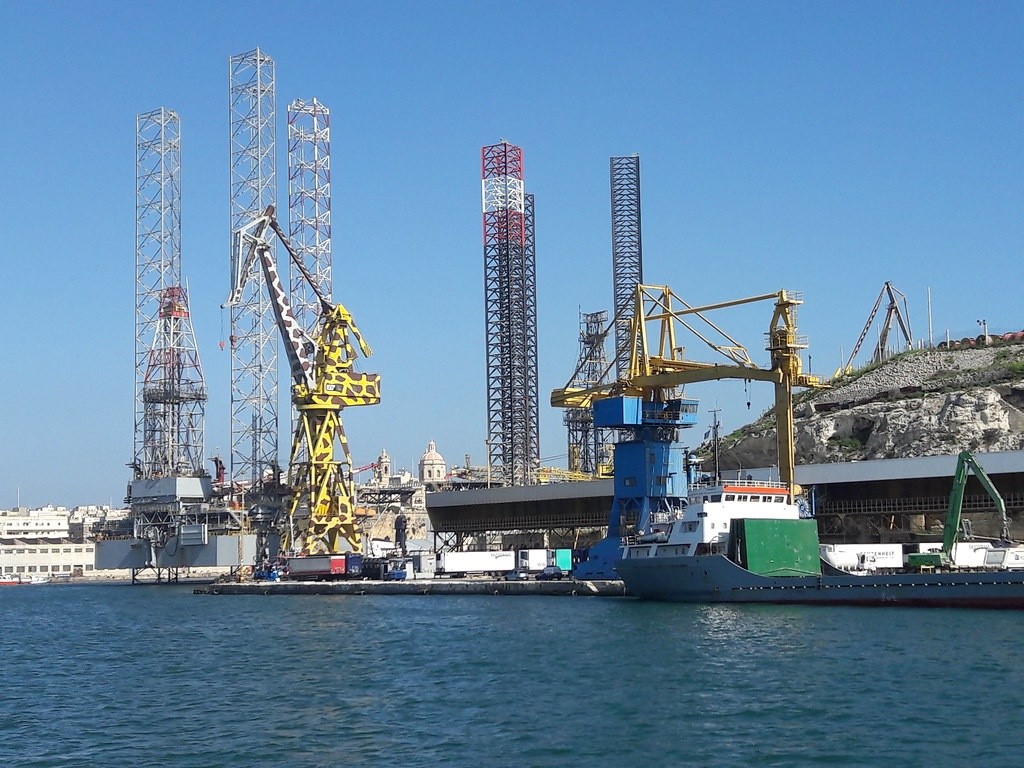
[[216, 202, 386, 574], [550, 285, 838, 500], [902, 449, 1015, 571]]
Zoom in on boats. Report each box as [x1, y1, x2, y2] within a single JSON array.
[[614, 473, 1024, 607]]
